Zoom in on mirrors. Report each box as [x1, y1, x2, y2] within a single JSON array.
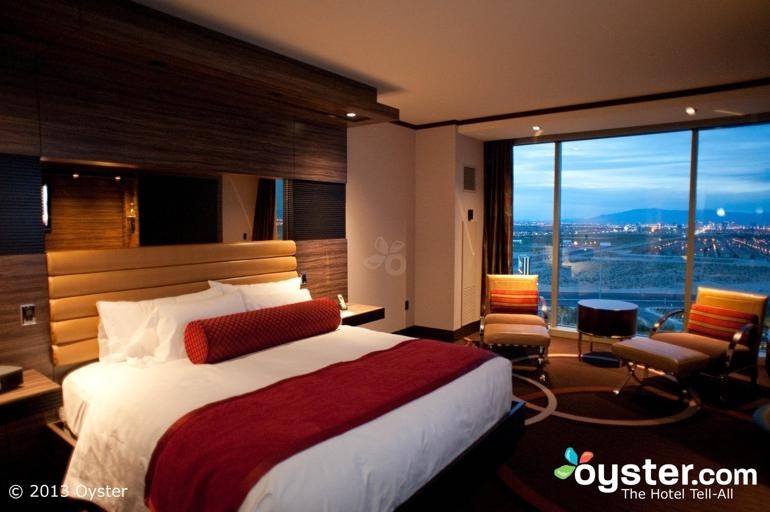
[[137, 170, 345, 245]]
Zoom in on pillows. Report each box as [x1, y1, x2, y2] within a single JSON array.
[[687, 304, 757, 341], [184, 296, 341, 364], [97, 284, 251, 362], [489, 289, 538, 315], [207, 277, 313, 329]]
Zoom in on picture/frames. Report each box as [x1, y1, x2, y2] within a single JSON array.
[[463, 166, 477, 190]]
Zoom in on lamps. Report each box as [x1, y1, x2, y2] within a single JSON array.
[[127, 201, 136, 236]]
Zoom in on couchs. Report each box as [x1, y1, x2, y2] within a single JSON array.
[[482, 274, 550, 369], [611, 287, 767, 419]]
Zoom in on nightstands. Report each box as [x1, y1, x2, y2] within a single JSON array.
[[335, 304, 385, 326], [0, 369, 63, 506]]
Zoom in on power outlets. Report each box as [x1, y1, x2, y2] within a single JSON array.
[[20, 304, 38, 329], [300, 271, 308, 285]]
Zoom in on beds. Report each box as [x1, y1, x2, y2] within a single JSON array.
[[576, 298, 637, 370], [48, 239, 511, 511]]
